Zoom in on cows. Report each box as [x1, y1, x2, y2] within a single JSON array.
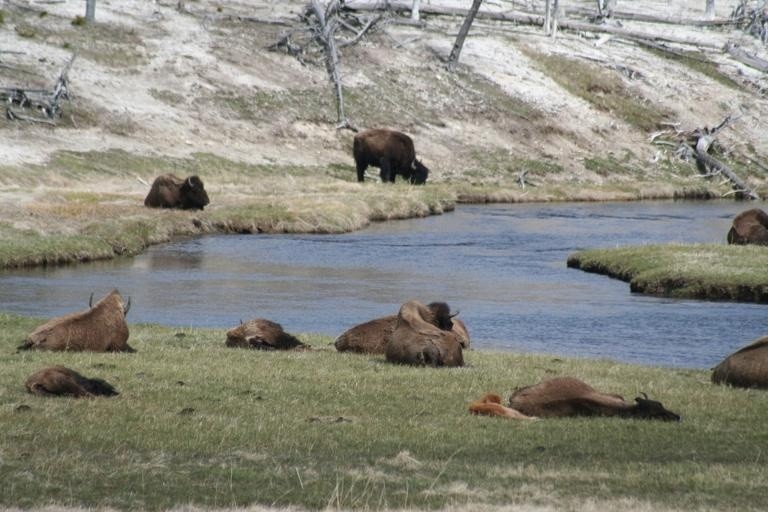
[[727, 208, 768, 247], [144, 172, 210, 212], [334, 298, 470, 368], [353, 129, 428, 186], [710, 335, 768, 390], [468, 375, 680, 422], [224, 317, 311, 351], [15, 286, 138, 354], [24, 364, 120, 401]]
